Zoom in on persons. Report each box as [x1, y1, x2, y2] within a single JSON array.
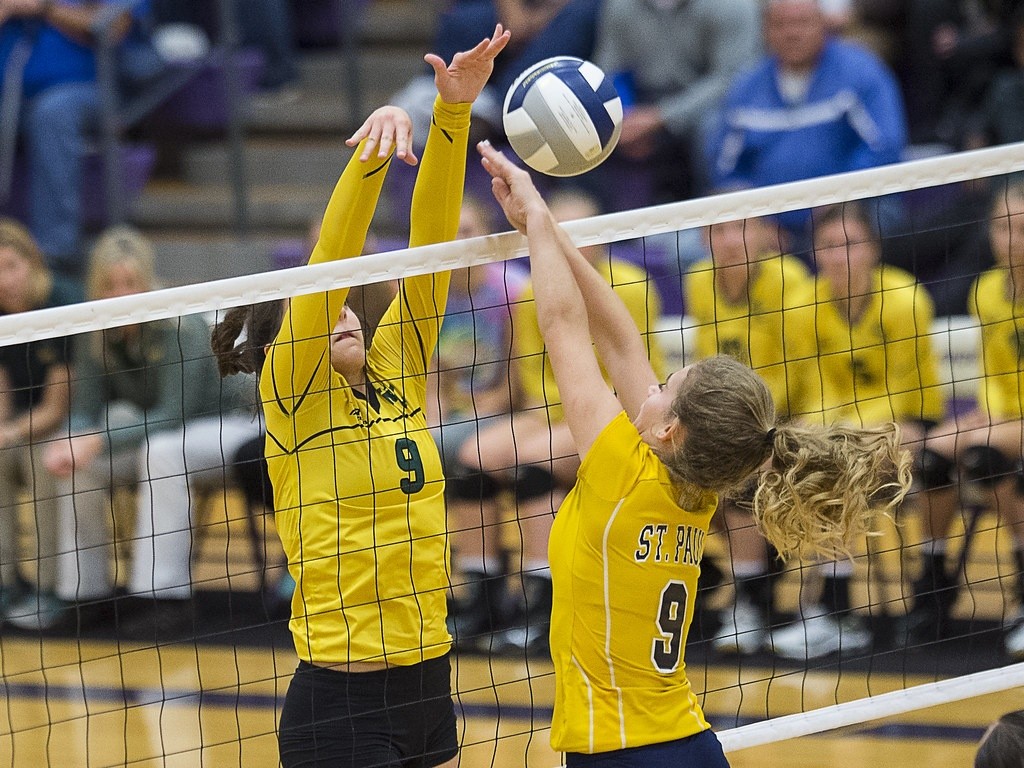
[[473, 130, 923, 768], [211, 22, 509, 768], [974, 708, 1024, 768], [716, 1, 912, 251], [2, 0, 1024, 669]]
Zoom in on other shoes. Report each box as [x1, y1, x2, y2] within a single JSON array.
[[474, 571, 552, 656], [8, 589, 61, 629], [710, 600, 767, 657], [894, 578, 960, 648], [763, 603, 871, 659], [443, 568, 501, 654], [49, 591, 196, 643]]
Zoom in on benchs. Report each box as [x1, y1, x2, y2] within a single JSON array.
[[0, 0, 996, 317]]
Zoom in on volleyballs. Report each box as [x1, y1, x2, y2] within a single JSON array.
[[501, 55, 624, 177]]
